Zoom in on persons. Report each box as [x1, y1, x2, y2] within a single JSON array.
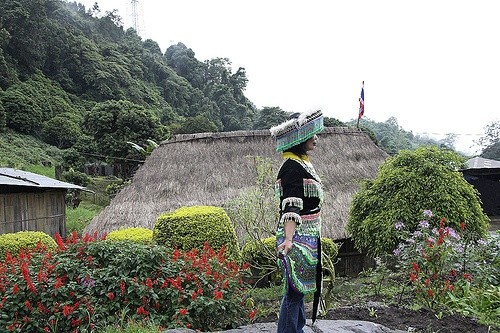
[[269, 107, 325, 333]]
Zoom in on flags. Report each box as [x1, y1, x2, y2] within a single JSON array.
[[358, 86, 365, 119]]
